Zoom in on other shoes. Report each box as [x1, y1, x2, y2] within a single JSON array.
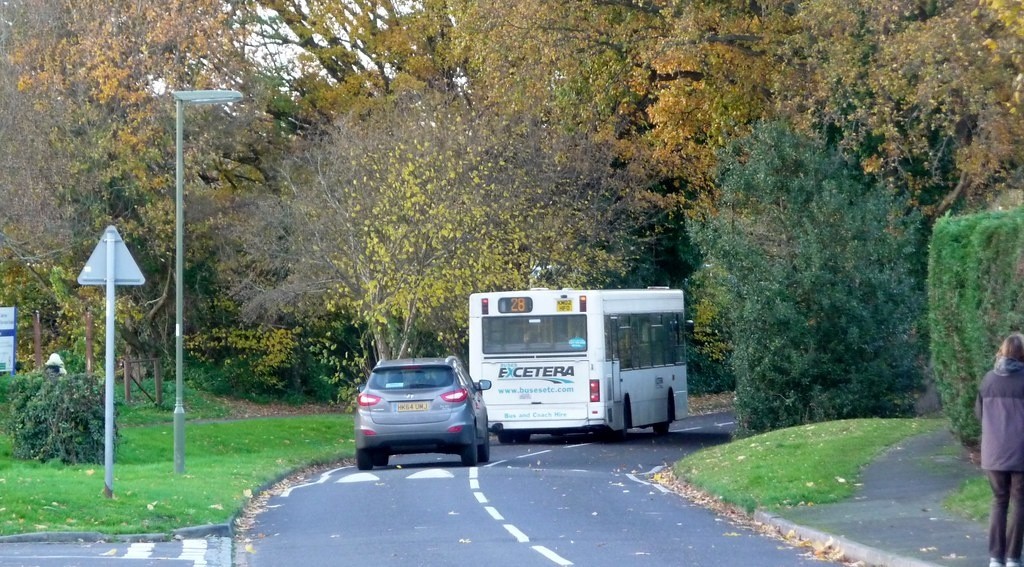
[[990, 557, 1006, 567], [1006, 557, 1023, 567]]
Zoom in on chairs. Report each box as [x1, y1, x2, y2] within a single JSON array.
[[484, 342, 584, 353], [388, 371, 447, 387]]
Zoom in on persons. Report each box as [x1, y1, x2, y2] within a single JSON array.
[[975, 334, 1024, 567], [45, 353, 67, 375]]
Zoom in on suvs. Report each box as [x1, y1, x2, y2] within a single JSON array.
[[352, 354, 492, 466]]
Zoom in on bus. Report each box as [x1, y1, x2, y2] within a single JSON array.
[[466, 285, 694, 444]]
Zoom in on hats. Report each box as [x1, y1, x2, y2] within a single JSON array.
[[49, 353, 60, 360]]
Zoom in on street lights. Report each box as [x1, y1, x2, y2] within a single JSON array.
[[172, 89, 244, 473]]
